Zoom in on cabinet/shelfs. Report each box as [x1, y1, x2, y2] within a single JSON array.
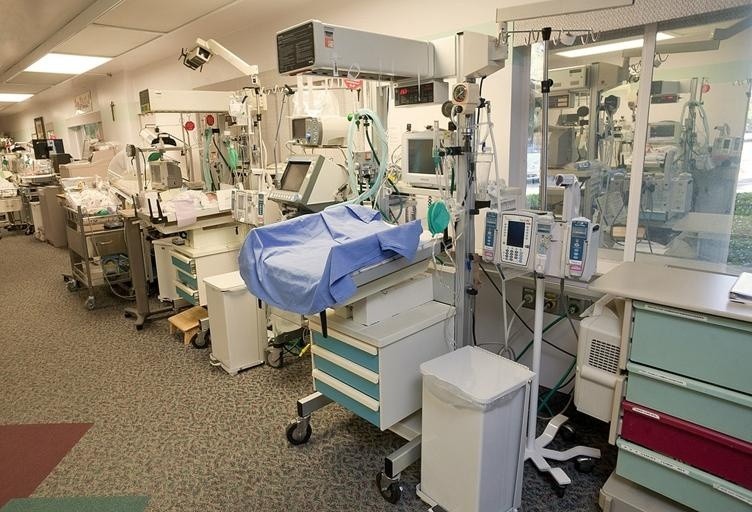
[[171, 245, 242, 306], [304, 302, 457, 432]]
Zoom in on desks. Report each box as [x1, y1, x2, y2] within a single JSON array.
[[587, 260, 752, 512]]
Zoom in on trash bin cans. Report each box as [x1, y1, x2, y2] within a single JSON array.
[[37, 186, 68, 247]]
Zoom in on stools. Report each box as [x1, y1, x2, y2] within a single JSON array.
[[168, 306, 210, 347]]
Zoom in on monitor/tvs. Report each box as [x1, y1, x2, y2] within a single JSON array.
[[149, 161, 182, 190], [402, 130, 446, 190], [527, 143, 541, 180], [647, 122, 681, 144], [267, 154, 346, 213], [292, 117, 323, 146]]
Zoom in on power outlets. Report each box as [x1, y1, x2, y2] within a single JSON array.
[[521, 287, 592, 321]]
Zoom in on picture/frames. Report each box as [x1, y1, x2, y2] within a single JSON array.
[[34, 117, 45, 140]]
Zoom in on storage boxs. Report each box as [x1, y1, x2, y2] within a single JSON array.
[[615, 299, 752, 512]]
